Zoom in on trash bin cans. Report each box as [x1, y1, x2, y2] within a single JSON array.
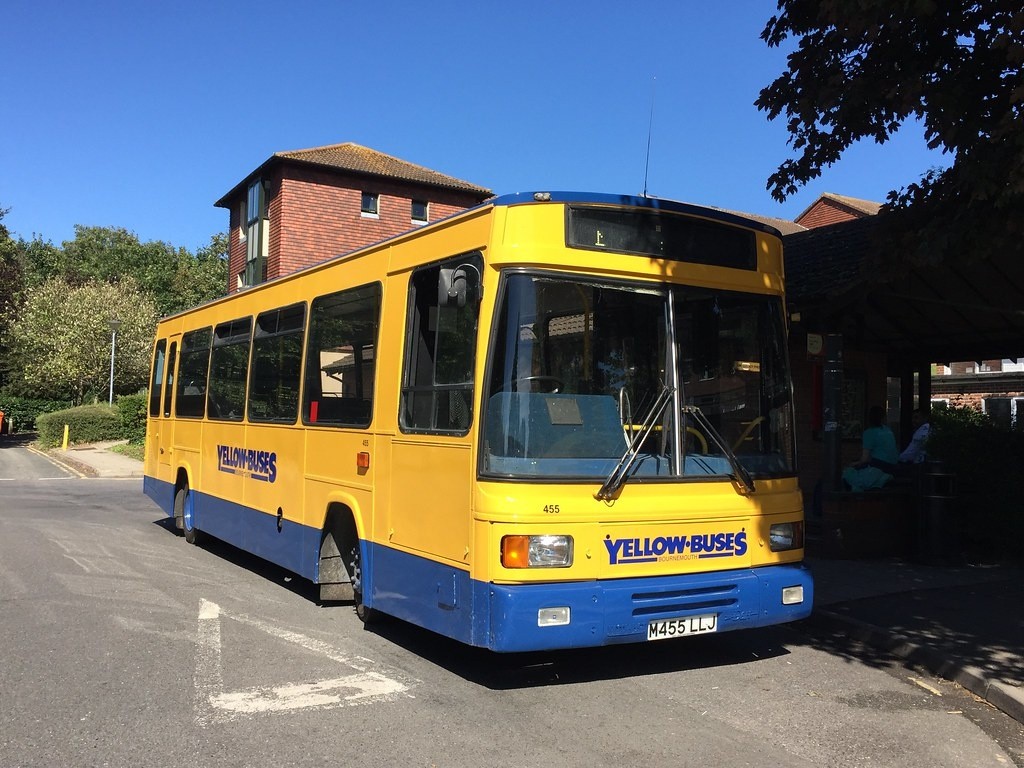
[[914, 457, 963, 568], [0, 411, 4, 433]]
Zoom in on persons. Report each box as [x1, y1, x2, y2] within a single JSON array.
[[849, 405, 897, 472], [898, 408, 932, 464]]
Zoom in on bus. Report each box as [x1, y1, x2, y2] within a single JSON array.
[[134, 190, 814, 654]]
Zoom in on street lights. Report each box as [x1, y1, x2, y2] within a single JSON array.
[[106, 321, 122, 406]]
